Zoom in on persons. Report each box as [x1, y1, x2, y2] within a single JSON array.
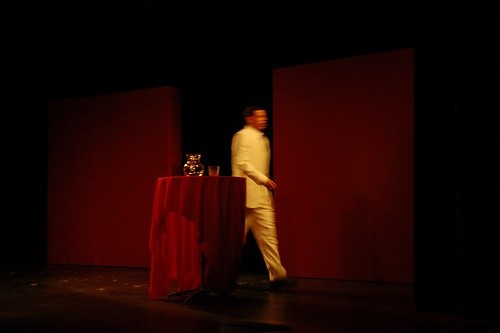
[[231, 105, 301, 289]]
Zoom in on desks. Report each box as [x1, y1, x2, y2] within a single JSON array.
[[150, 176, 246, 305]]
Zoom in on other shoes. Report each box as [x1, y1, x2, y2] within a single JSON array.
[[272, 277, 298, 290], [234, 278, 249, 287]]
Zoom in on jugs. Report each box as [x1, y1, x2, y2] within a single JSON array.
[[183, 154, 203, 176]]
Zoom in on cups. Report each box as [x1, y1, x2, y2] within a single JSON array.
[[208, 166, 220, 176]]
